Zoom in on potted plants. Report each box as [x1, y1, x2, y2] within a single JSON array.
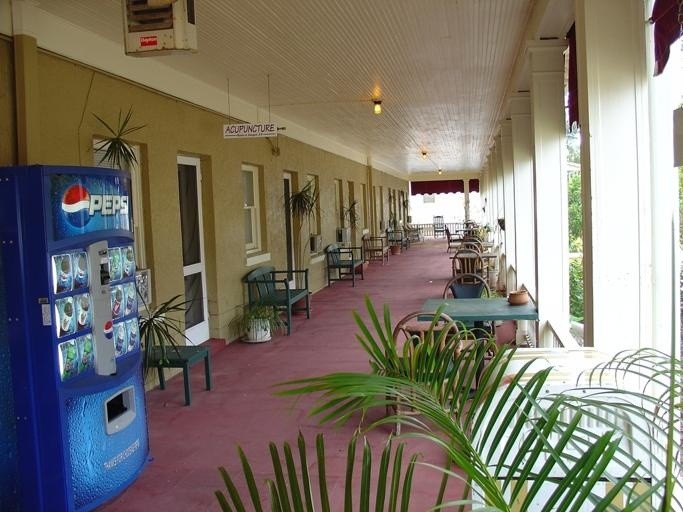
[[228, 295, 286, 343], [390, 230, 401, 255]]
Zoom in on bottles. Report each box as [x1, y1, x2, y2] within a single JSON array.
[[109, 245, 138, 355], [56, 255, 91, 380]]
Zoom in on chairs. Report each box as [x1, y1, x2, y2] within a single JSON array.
[[445, 220, 497, 281], [362, 233, 390, 265], [385, 311, 463, 436], [241, 264, 311, 336], [432, 216, 446, 239], [326, 244, 365, 288], [386, 227, 410, 251], [442, 273, 496, 360]]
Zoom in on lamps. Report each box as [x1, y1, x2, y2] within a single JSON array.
[[373, 100, 382, 114], [439, 170, 442, 175], [422, 153, 426, 159]]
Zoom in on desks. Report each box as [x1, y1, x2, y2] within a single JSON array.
[[417, 296, 538, 406], [468, 345, 683, 512], [141, 345, 212, 406]]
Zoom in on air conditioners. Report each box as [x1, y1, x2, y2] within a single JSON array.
[[310, 233, 323, 254], [336, 227, 351, 242], [407, 216, 412, 223]]
